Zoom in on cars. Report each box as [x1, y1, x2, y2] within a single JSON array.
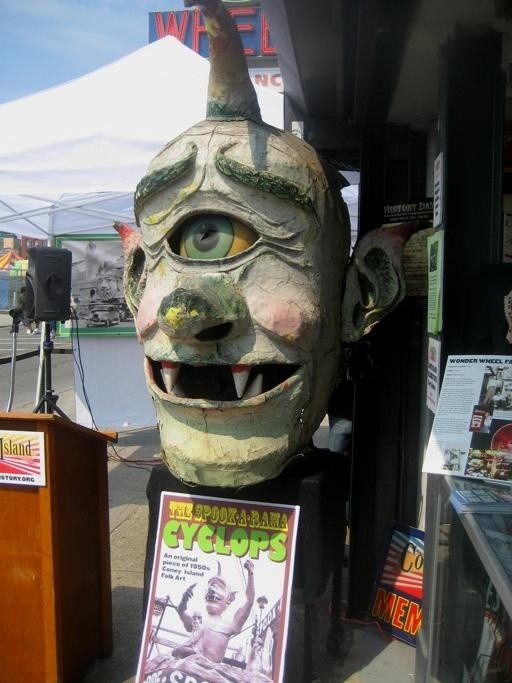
[[85, 304, 121, 329]]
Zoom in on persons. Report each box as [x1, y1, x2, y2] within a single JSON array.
[[172, 610, 205, 658]]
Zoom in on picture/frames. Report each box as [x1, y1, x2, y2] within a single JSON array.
[[53, 232, 136, 338]]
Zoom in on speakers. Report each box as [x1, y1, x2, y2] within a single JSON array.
[[24, 247, 72, 321]]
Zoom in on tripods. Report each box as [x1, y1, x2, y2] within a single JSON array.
[[32, 321, 73, 423]]
